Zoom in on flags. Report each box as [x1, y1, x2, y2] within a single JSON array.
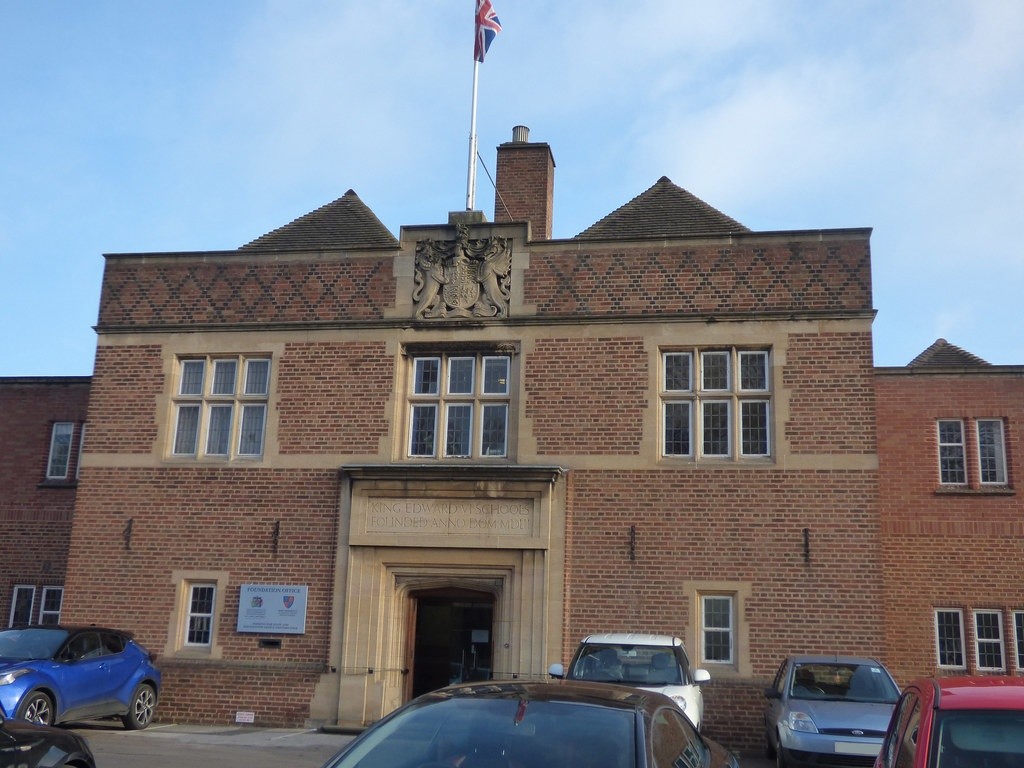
[[475, 0, 502, 63]]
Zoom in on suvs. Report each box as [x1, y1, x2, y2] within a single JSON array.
[[0, 622, 163, 729], [548, 633, 712, 734]]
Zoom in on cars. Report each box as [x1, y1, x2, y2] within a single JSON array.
[[762, 655, 902, 768], [871, 674, 1024, 768], [0, 697, 99, 768], [320, 677, 745, 768]]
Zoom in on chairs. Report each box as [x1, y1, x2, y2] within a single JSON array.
[[48, 638, 69, 657], [464, 717, 513, 768], [796, 669, 825, 695], [647, 652, 677, 682], [841, 675, 874, 697], [592, 648, 625, 679]]
